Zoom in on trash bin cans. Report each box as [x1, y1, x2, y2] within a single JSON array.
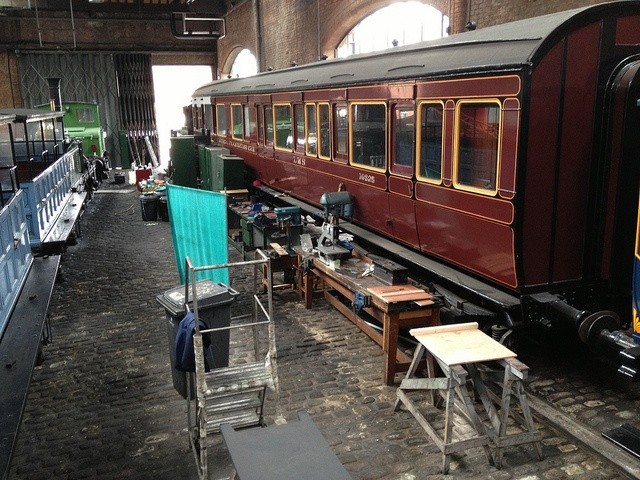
[[159, 196, 169, 221], [139, 192, 159, 221], [155, 280, 239, 400]]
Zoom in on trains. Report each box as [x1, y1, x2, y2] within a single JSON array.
[[184, 0, 640, 389]]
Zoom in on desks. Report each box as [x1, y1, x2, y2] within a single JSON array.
[[291, 242, 439, 386]]
